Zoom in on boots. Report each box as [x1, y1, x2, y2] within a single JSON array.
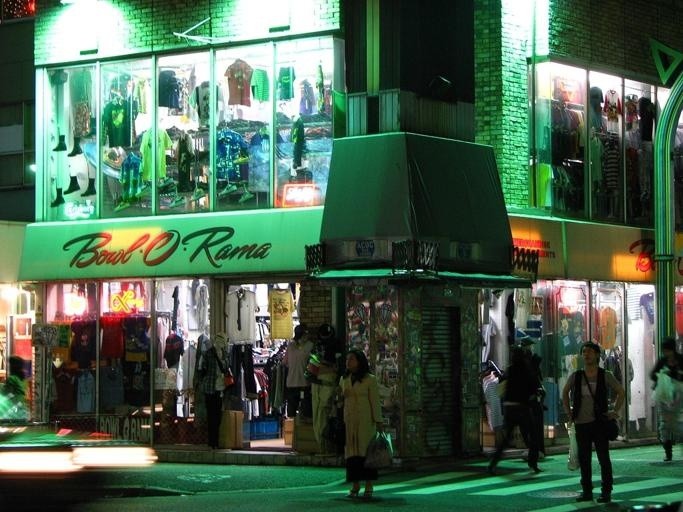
[[596, 480, 613, 503], [662, 442, 673, 461], [574, 478, 593, 503]]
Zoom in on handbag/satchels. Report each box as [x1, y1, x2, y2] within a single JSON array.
[[562, 419, 581, 471], [597, 415, 620, 443], [223, 366, 235, 386], [364, 425, 395, 471]]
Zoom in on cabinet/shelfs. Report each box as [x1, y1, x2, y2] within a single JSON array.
[[112, 120, 333, 217]]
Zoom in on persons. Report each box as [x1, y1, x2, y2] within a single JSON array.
[[485, 348, 548, 475], [335, 347, 388, 500], [560, 341, 625, 504], [199, 333, 235, 453], [281, 324, 314, 419], [552, 84, 579, 130], [0, 355, 28, 420], [305, 333, 341, 459], [647, 337, 682, 462]]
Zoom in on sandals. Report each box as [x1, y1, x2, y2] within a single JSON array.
[[345, 484, 373, 503]]
